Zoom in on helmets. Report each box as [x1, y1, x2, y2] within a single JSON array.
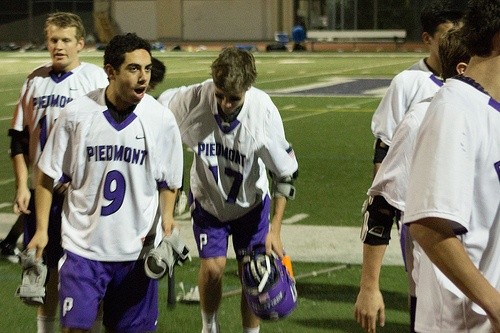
[[237, 252, 299, 323]]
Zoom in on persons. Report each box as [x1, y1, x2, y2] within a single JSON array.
[[26, 32, 184, 333], [0, 57, 165, 266], [156, 46, 299, 333], [353, 0, 500, 333], [7, 13, 109, 333]]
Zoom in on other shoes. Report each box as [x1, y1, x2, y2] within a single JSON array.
[[0, 237, 23, 265]]
